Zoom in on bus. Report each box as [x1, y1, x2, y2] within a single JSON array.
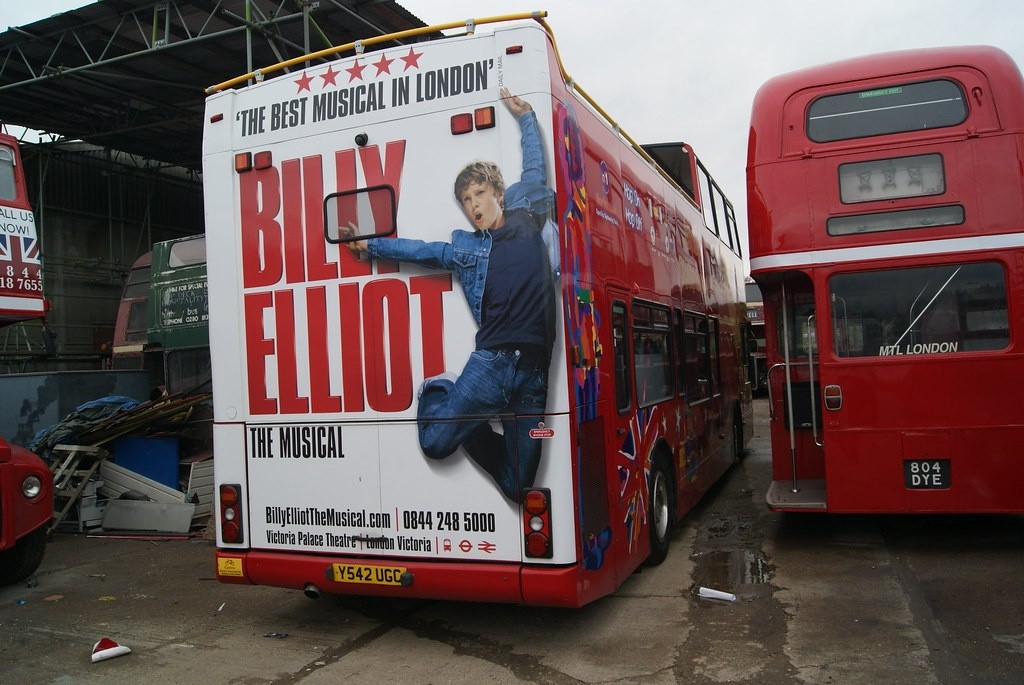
[[194, 7, 759, 612], [745, 43, 1024, 518]]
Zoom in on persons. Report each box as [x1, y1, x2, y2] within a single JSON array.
[[335, 87, 556, 504]]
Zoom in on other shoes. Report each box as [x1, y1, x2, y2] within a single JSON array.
[[417, 371, 458, 397]]
